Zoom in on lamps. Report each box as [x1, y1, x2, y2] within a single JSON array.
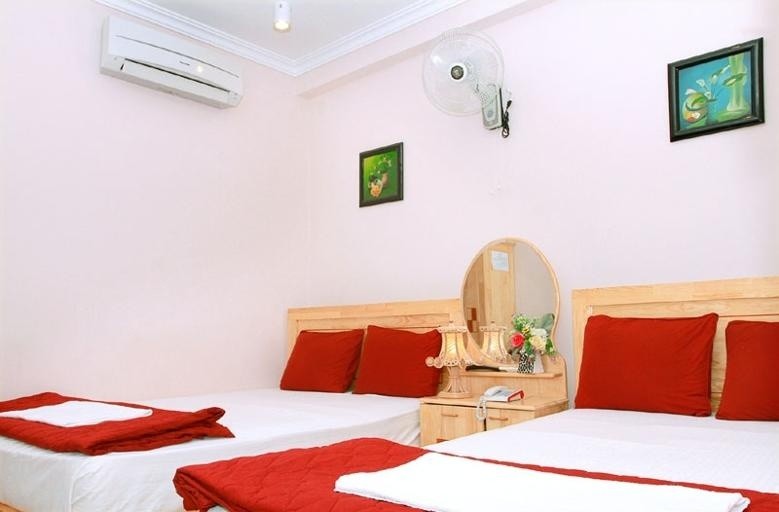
[[424, 321, 479, 399], [477, 320, 509, 363], [271, 3, 292, 35]]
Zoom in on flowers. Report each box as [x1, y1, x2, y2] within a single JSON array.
[[509, 311, 559, 357]]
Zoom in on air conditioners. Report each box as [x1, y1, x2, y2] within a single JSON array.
[[101, 19, 246, 112]]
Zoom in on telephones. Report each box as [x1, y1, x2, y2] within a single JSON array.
[[476, 385, 524, 421]]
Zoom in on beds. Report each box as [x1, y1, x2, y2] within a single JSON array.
[[173, 277, 779, 512], [1, 299, 471, 512]]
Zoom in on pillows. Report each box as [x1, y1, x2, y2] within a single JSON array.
[[420, 29, 512, 137], [350, 323, 443, 398], [574, 304, 717, 418], [719, 315, 770, 419], [278, 322, 354, 396]]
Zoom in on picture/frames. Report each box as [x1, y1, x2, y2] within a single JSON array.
[[667, 36, 764, 145], [357, 142, 405, 209]]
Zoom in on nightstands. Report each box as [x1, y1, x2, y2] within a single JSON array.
[[417, 237, 568, 445]]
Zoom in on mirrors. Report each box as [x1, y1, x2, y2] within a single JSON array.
[[462, 241, 558, 362]]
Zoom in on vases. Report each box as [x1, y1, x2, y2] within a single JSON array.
[[515, 355, 536, 376]]
